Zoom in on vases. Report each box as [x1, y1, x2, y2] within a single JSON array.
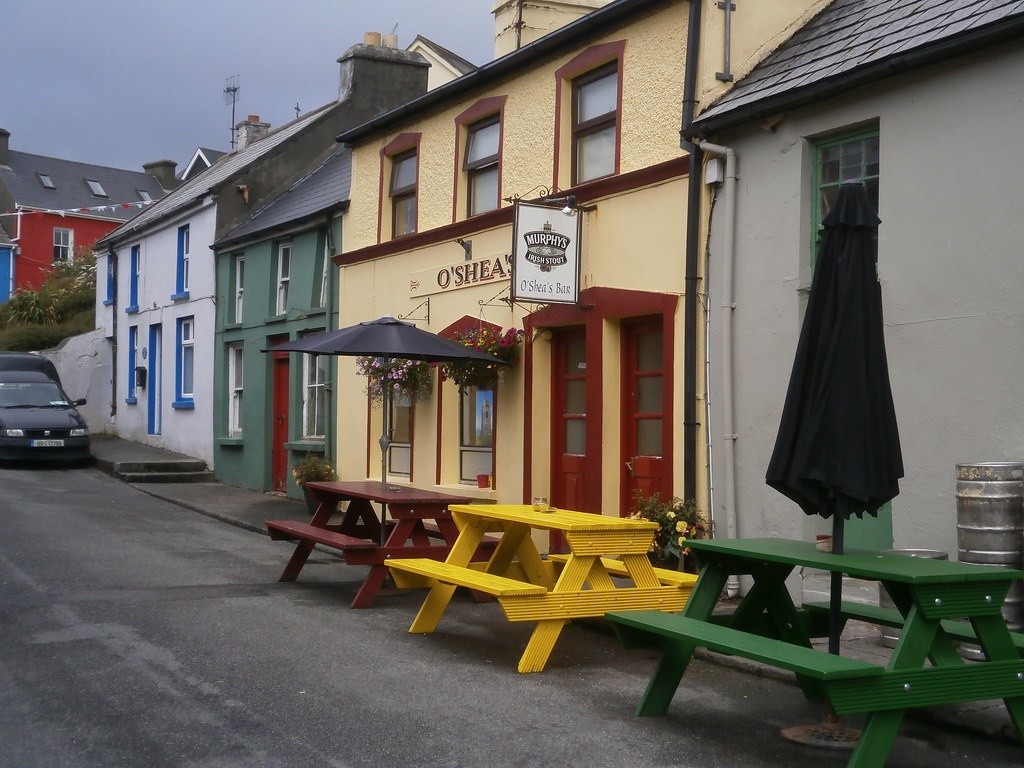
[[394, 388, 412, 407], [302, 487, 323, 514], [477, 368, 498, 390]]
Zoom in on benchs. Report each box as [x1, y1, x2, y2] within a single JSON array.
[[603, 608, 886, 681], [801, 599, 1024, 656], [386, 518, 502, 545], [266, 520, 378, 550], [384, 557, 547, 596], [547, 553, 700, 587]]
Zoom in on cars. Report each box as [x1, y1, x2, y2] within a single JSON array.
[[0, 351, 62, 390], [0, 368, 93, 468]]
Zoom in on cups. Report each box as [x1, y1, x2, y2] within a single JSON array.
[[477, 475, 489, 488]]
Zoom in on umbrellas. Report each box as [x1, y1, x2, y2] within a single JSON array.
[[259, 317, 504, 481], [760, 179, 907, 650]]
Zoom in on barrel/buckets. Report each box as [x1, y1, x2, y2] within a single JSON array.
[[880, 549, 950, 649], [955, 460, 1024, 661]]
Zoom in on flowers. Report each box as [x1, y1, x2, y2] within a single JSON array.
[[354, 354, 432, 410], [292, 453, 341, 489], [625, 488, 710, 571], [432, 326, 526, 396]]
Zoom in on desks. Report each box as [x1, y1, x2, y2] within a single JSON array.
[[683, 535, 1024, 585], [304, 480, 469, 505], [448, 504, 660, 531]]
